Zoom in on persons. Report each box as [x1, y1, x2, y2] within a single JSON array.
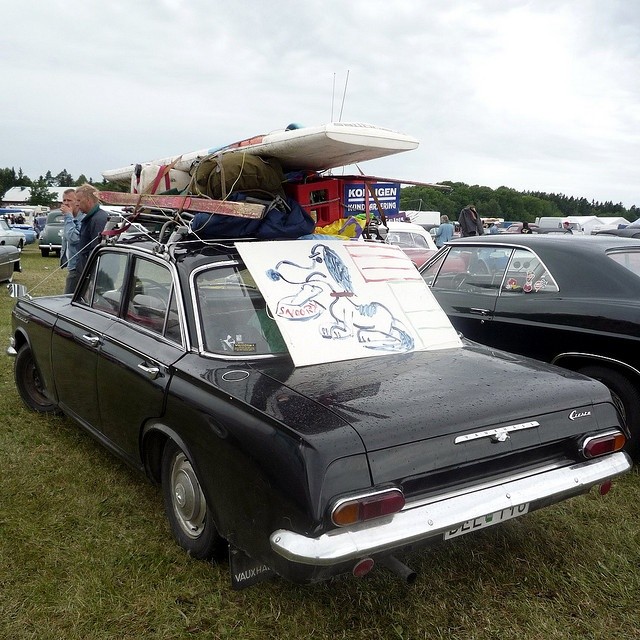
[[521, 221, 532, 234], [490, 221, 502, 235], [2, 213, 26, 224], [458, 206, 486, 236], [433, 215, 454, 250], [71, 184, 110, 294], [60, 189, 88, 295], [563, 221, 569, 228]]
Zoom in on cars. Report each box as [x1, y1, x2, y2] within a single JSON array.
[[371, 222, 438, 268], [11, 238, 634, 589], [411, 234, 640, 466], [506, 224, 539, 233], [39, 209, 64, 257], [0, 219, 26, 249], [0, 244, 21, 283]]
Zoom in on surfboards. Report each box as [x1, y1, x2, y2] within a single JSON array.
[[101, 122, 420, 173]]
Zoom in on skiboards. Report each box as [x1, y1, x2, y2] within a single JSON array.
[[93, 191, 265, 221]]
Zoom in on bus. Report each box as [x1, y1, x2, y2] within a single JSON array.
[[5, 205, 50, 231]]
